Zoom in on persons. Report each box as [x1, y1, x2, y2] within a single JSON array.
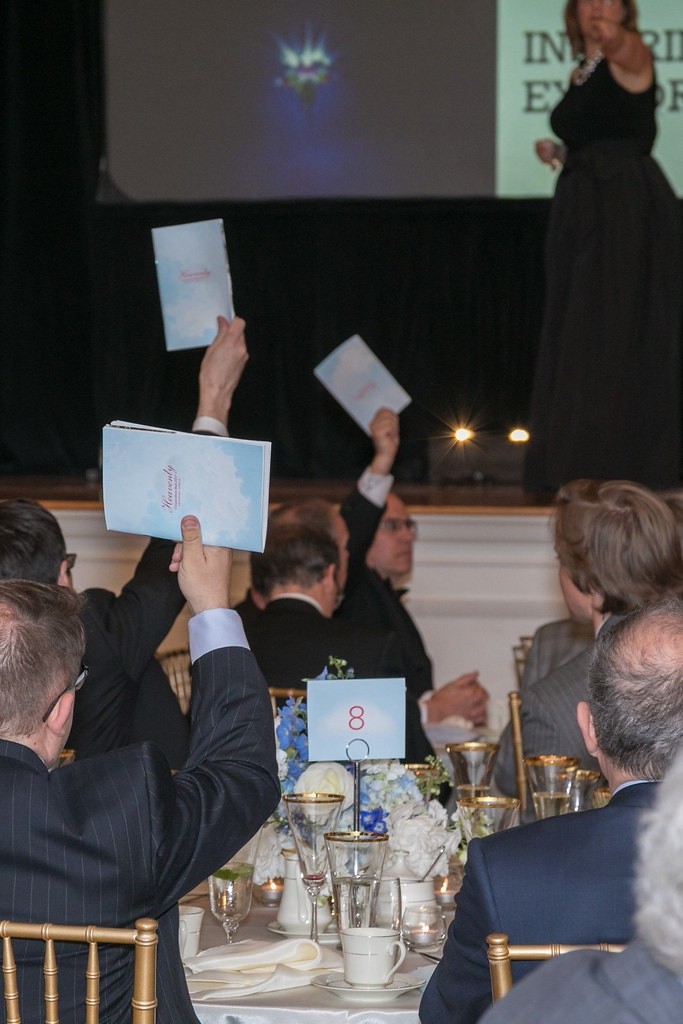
[[526, 0, 683, 504], [418, 598, 683, 1024], [248, 528, 451, 808], [341, 406, 418, 691], [479, 753, 683, 1024], [0, 516, 282, 1024], [232, 501, 489, 731], [0, 317, 249, 771], [485, 481, 683, 826], [520, 490, 683, 694]]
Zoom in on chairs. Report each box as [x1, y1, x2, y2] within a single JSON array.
[[0, 916, 160, 1024], [485, 932, 637, 1001]]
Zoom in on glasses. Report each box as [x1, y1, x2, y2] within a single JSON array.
[[43, 658, 90, 722], [381, 515, 418, 536], [59, 551, 78, 570]]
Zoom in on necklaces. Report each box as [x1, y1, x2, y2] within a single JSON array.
[[571, 49, 605, 85]]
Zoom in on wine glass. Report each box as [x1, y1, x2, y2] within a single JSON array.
[[282, 792, 345, 946], [207, 862, 256, 945]]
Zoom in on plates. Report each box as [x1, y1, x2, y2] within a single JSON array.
[[268, 922, 341, 936], [310, 972, 427, 1001]]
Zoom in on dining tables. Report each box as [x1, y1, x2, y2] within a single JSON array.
[[174, 891, 455, 1024]]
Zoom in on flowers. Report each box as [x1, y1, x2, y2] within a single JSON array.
[[276, 654, 499, 867]]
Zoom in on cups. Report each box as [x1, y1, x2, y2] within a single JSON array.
[[398, 876, 440, 928], [400, 904, 447, 955], [178, 905, 205, 961], [323, 831, 390, 952], [403, 742, 611, 840], [339, 927, 407, 990], [348, 874, 402, 936]]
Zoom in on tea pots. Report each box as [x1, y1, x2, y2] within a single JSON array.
[[276, 852, 334, 939]]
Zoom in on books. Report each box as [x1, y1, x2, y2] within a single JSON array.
[[151, 218, 235, 352], [314, 333, 411, 437], [101, 419, 271, 554]]
